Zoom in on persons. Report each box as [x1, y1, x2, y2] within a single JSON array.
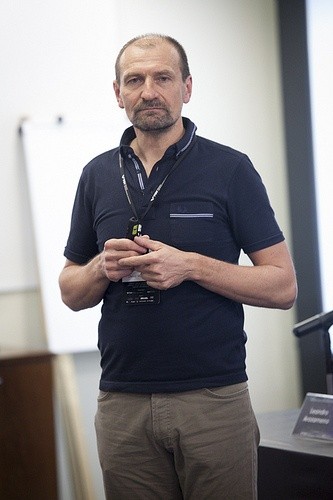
[[58, 34, 299, 500]]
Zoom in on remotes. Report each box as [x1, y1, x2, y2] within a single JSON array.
[[125, 217, 139, 240]]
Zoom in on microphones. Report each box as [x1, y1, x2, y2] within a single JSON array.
[[293, 312, 332, 337]]
